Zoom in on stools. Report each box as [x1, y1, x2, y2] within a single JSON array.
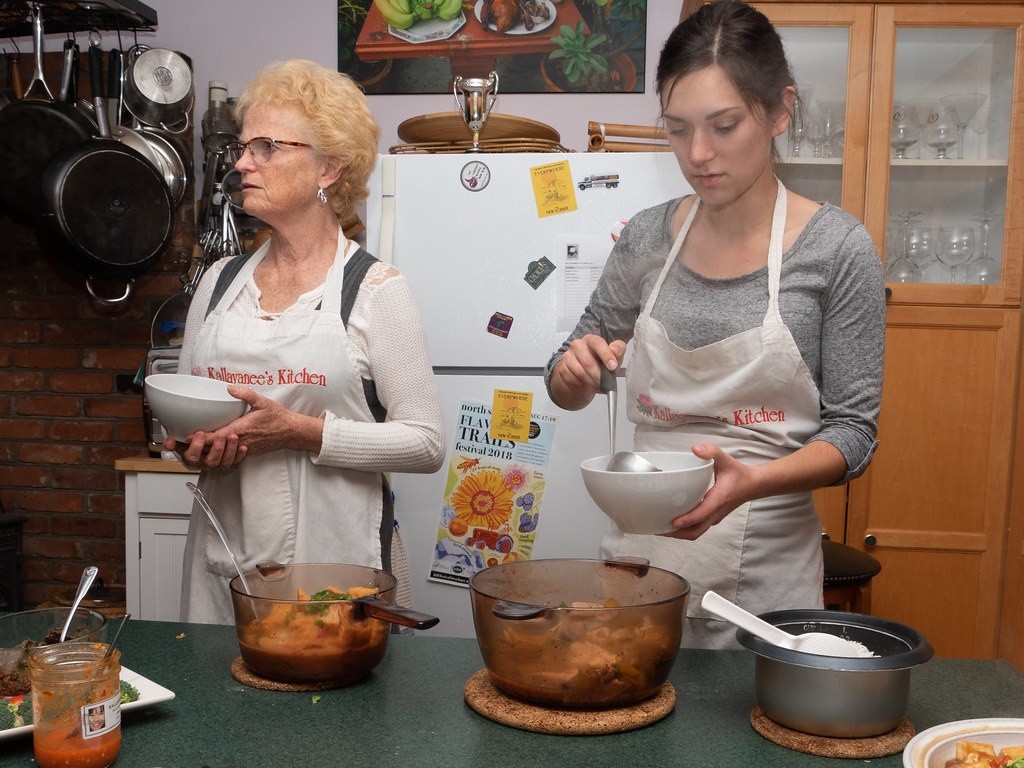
[[821, 539, 882, 616]]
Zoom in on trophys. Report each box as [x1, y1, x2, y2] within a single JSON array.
[[453, 72, 499, 152]]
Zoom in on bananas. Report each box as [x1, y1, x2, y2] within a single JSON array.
[[373, 0, 463, 28]]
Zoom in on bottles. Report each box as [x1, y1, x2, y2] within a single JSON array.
[[29, 643, 123, 768]]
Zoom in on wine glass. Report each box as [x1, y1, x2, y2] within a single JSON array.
[[453, 70, 499, 152], [787, 83, 1001, 286]]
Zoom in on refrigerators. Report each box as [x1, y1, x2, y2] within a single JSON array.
[[357, 148, 693, 637]]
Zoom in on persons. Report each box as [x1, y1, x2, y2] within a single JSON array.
[[545, 1, 885, 649], [164, 58, 444, 626]]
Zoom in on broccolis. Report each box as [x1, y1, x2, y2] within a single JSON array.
[[0, 679, 140, 733]]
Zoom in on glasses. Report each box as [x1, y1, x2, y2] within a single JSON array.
[[224, 134, 313, 166]]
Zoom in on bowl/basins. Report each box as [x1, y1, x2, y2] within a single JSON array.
[[0, 605, 109, 699], [579, 451, 714, 535], [902, 717, 1024, 768], [144, 373, 247, 443]]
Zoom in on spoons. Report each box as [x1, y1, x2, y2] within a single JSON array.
[[697, 590, 858, 657]]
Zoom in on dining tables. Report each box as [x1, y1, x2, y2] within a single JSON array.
[[354, 0, 592, 92]]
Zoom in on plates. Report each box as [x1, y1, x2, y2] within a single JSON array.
[[474, 0, 557, 35], [0, 665, 177, 740], [387, 9, 467, 45]]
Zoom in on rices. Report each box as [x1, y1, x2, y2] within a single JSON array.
[[847, 641, 882, 657]]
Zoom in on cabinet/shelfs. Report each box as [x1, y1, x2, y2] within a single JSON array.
[[113, 453, 202, 621], [679, 0, 1024, 660]]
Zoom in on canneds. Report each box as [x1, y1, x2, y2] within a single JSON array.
[[29, 641, 122, 768]]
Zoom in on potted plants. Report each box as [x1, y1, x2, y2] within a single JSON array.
[[338, 0, 394, 94], [540, 0, 645, 93]]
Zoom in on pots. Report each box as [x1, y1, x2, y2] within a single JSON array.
[[229, 561, 441, 685], [0, 9, 195, 308], [734, 608, 936, 739], [469, 555, 691, 713]]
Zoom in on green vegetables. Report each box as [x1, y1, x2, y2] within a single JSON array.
[[303, 590, 352, 627]]
